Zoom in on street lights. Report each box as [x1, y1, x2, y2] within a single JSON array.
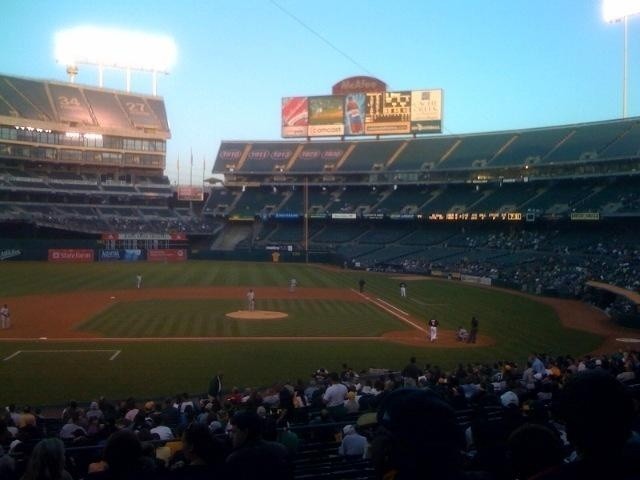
[[598, 0, 639, 121]]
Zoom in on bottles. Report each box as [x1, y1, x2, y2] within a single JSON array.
[[345, 95, 364, 135]]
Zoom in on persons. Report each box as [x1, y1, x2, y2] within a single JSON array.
[[2, 347, 639, 479], [1, 160, 219, 235], [455, 315, 479, 344], [342, 159, 639, 331], [290, 277, 297, 290], [247, 289, 256, 312], [428, 316, 440, 342], [1, 304, 10, 329], [136, 273, 144, 289]]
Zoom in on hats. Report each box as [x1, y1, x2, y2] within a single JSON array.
[[500, 391, 519, 408]]
